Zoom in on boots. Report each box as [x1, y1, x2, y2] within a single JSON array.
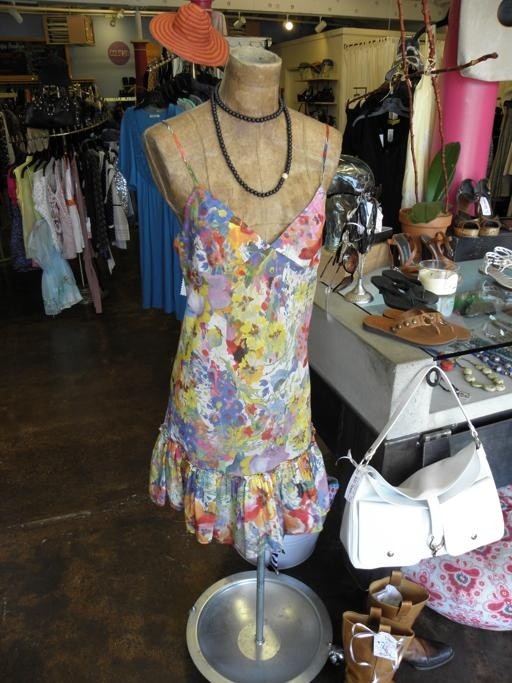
[[336, 573, 455, 681]]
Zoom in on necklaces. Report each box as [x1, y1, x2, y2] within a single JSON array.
[[427, 320, 512, 401], [211, 81, 292, 197]]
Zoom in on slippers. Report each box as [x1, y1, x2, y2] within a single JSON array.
[[364, 267, 472, 348]]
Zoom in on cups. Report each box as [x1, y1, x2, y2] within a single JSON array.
[[418, 260, 460, 295]]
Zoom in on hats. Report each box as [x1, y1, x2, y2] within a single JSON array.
[[147, 2, 231, 70]]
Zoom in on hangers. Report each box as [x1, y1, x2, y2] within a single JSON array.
[[347, 37, 423, 128], [0, 55, 214, 178]]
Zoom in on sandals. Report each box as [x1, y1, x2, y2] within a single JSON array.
[[386, 231, 461, 279], [479, 245, 512, 290], [453, 177, 501, 237]]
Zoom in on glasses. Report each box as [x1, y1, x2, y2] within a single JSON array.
[[338, 222, 360, 281]]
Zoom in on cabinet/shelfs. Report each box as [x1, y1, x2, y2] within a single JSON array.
[[297, 78, 338, 128], [308, 241, 511, 592]]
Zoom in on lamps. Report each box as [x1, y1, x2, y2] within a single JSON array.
[[0, 6, 331, 34]]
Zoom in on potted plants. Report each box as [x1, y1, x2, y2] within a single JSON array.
[[399, 141, 461, 239]]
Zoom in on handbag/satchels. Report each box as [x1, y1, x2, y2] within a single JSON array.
[[336, 364, 507, 572], [21, 81, 78, 129]]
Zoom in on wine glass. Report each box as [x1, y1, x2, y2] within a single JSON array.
[[346, 198, 375, 303]]
